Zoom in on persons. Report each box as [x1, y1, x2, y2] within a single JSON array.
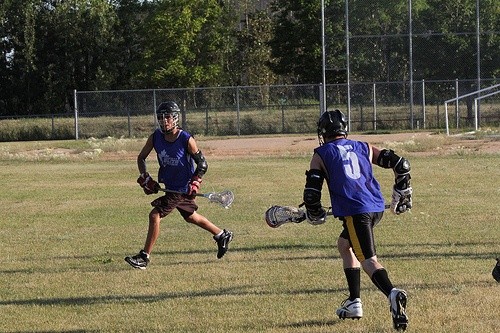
[[124, 101, 234, 270], [303, 109, 413, 332]]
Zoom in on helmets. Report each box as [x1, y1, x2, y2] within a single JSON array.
[[156, 102, 181, 135], [317, 109, 348, 144]]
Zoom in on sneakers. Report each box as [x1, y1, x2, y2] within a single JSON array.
[[389, 288, 408, 333], [125, 250, 149, 269], [336, 293, 362, 320], [213, 229, 233, 258]]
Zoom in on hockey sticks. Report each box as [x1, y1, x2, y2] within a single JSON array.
[[157, 187, 235, 208], [264, 202, 412, 228]]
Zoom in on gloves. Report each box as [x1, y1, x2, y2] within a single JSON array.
[[390, 188, 413, 215], [185, 177, 202, 199], [305, 209, 327, 225], [137, 173, 161, 195]]
